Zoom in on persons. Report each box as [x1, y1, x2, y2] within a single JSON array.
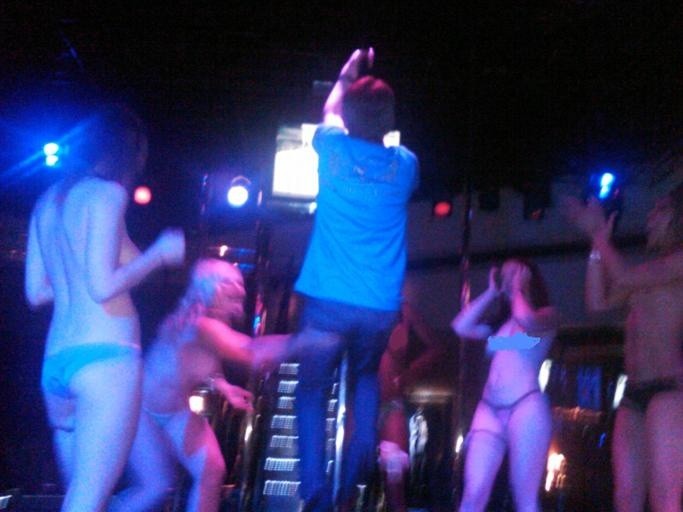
[[283, 42, 424, 510], [555, 184, 682, 512], [342, 298, 448, 511], [109, 255, 292, 511], [20, 103, 188, 510], [447, 254, 565, 511]]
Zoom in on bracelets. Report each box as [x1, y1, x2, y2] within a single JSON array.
[[587, 249, 603, 263]]
